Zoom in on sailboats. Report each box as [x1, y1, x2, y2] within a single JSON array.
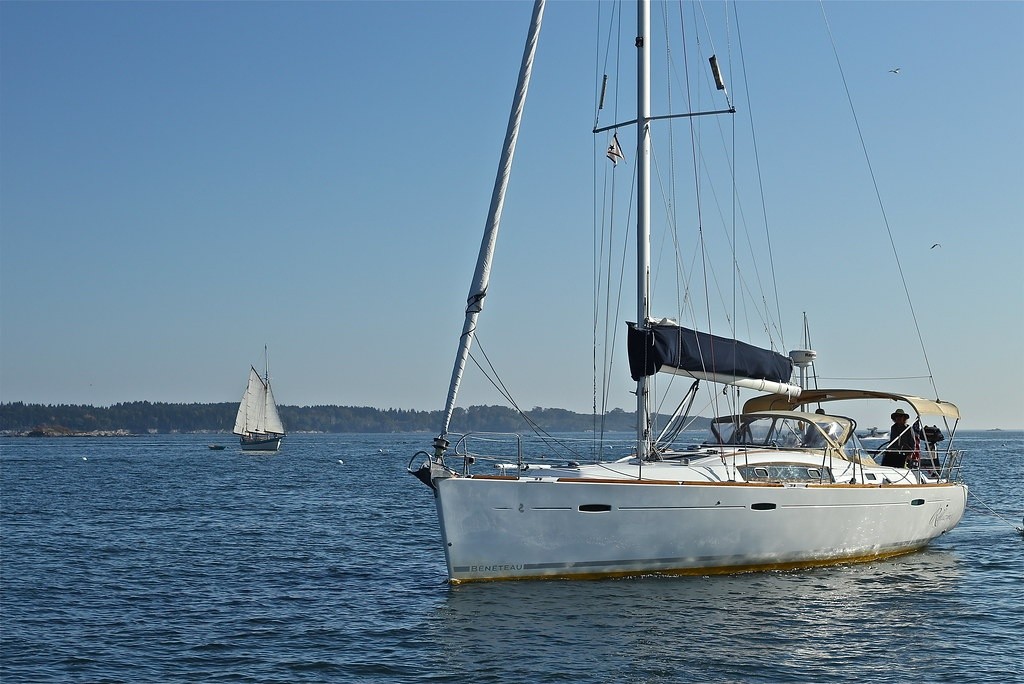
[[232, 342, 287, 451], [403, 0, 970, 585]]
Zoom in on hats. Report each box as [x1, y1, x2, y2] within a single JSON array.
[[815, 409, 825, 415], [891, 409, 909, 421]]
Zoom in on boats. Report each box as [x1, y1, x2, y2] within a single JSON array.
[[207, 444, 226, 450]]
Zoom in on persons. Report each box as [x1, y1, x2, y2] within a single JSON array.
[[889, 409, 916, 468], [803, 408, 838, 449], [795, 421, 805, 438]]
